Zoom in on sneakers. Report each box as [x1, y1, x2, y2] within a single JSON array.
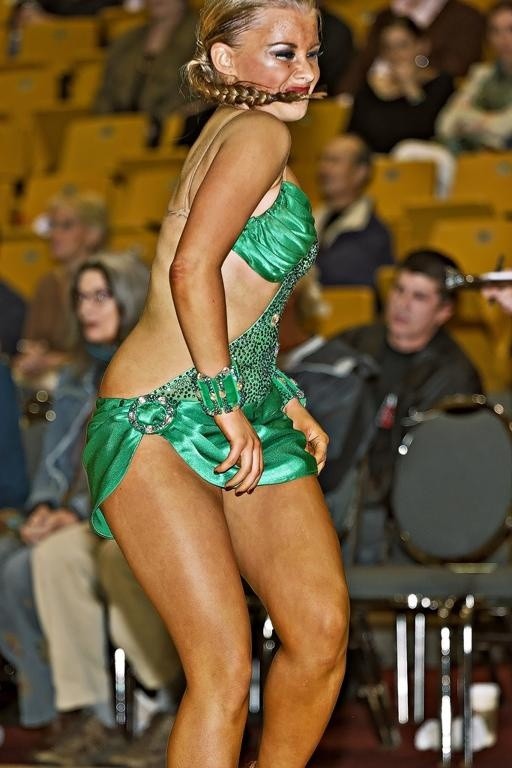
[[28, 709, 175, 768]]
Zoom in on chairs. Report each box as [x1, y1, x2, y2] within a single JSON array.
[[0, 1, 512, 768]]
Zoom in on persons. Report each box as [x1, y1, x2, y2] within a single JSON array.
[[0, 0, 511, 767]]
